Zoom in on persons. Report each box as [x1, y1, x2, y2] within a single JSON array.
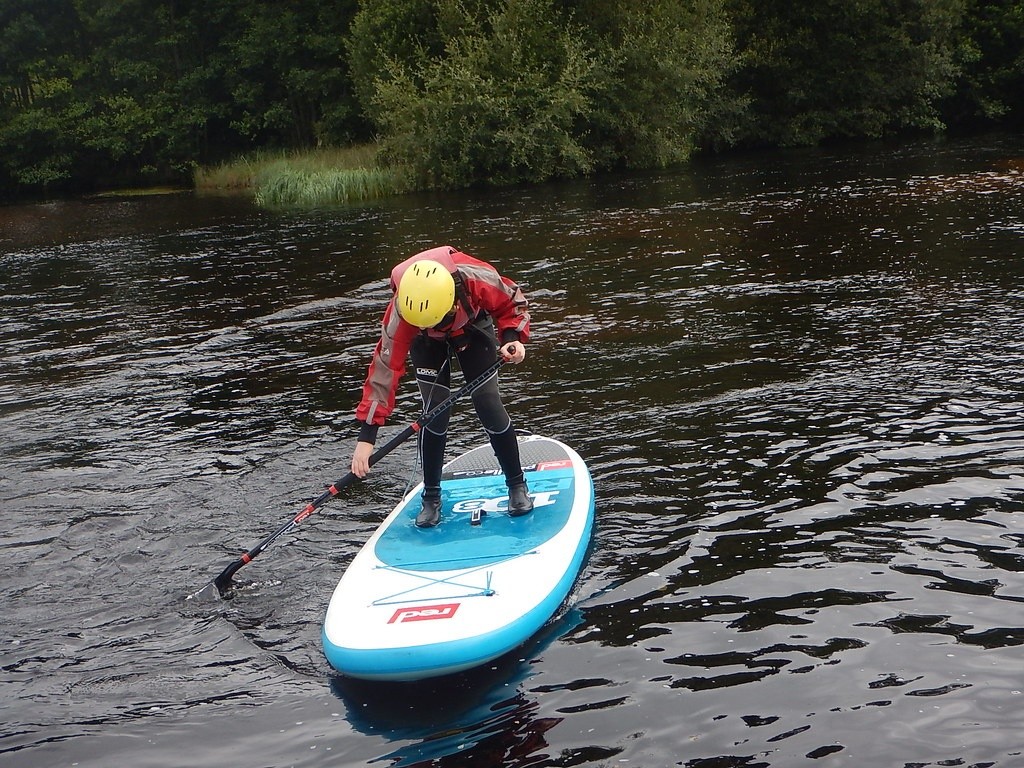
[[351, 245, 534, 528]]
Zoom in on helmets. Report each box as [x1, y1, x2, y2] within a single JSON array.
[[398, 259, 455, 328]]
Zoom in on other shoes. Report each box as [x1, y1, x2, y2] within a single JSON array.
[[508, 482, 533, 515], [415, 494, 443, 526]]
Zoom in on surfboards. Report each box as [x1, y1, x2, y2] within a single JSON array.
[[321, 430, 599, 685]]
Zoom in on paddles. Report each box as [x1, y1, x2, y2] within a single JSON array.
[[207, 342, 520, 598]]
